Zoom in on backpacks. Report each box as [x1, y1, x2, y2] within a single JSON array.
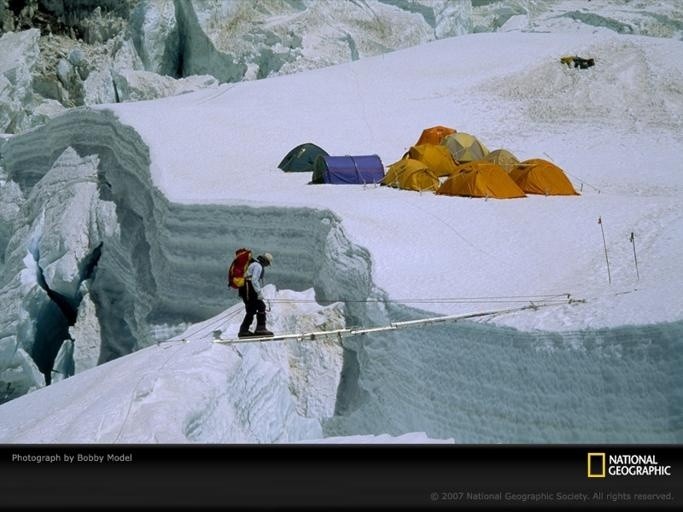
[[228, 248, 257, 288]]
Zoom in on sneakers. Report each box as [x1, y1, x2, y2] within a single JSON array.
[[255, 327, 273, 335], [239, 330, 253, 336]]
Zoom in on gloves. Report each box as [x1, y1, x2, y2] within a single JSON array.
[[258, 294, 263, 299]]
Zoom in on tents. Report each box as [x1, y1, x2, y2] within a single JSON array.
[[277, 125, 581, 199]]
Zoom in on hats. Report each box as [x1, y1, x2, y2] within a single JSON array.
[[263, 253, 273, 266]]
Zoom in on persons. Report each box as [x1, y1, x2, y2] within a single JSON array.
[[238, 253, 275, 337]]
